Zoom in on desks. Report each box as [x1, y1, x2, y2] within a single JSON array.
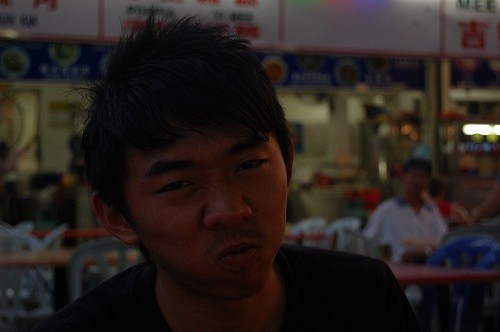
[[0, 245, 143, 321], [388, 261, 500, 332]]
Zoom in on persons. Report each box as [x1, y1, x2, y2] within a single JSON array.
[[470, 177, 498, 226], [425, 176, 472, 228], [39, 4, 420, 331], [0, 138, 40, 227], [65, 133, 85, 183], [364, 158, 448, 258]]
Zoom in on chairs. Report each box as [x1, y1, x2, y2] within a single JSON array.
[[0, 222, 70, 251], [294, 216, 360, 253]]
